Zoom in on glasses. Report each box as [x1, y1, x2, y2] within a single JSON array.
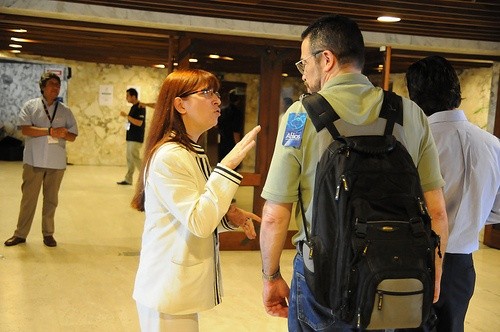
[[179, 87, 221, 99], [295, 49, 335, 76]]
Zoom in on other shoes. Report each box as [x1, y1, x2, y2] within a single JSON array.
[[117, 180, 132, 186]]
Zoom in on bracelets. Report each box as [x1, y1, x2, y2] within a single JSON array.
[[48, 127, 53, 136], [261, 266, 281, 281]]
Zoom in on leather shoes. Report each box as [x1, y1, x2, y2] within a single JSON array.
[[43, 235, 57, 247], [5, 235, 26, 246]]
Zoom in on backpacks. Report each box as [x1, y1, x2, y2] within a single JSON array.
[[295, 89, 442, 332]]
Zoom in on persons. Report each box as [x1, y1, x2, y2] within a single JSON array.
[[259, 15, 449, 332], [117, 88, 147, 185], [4, 71, 78, 246], [138, 102, 156, 108], [132, 68, 263, 331], [404, 54, 500, 332]]
